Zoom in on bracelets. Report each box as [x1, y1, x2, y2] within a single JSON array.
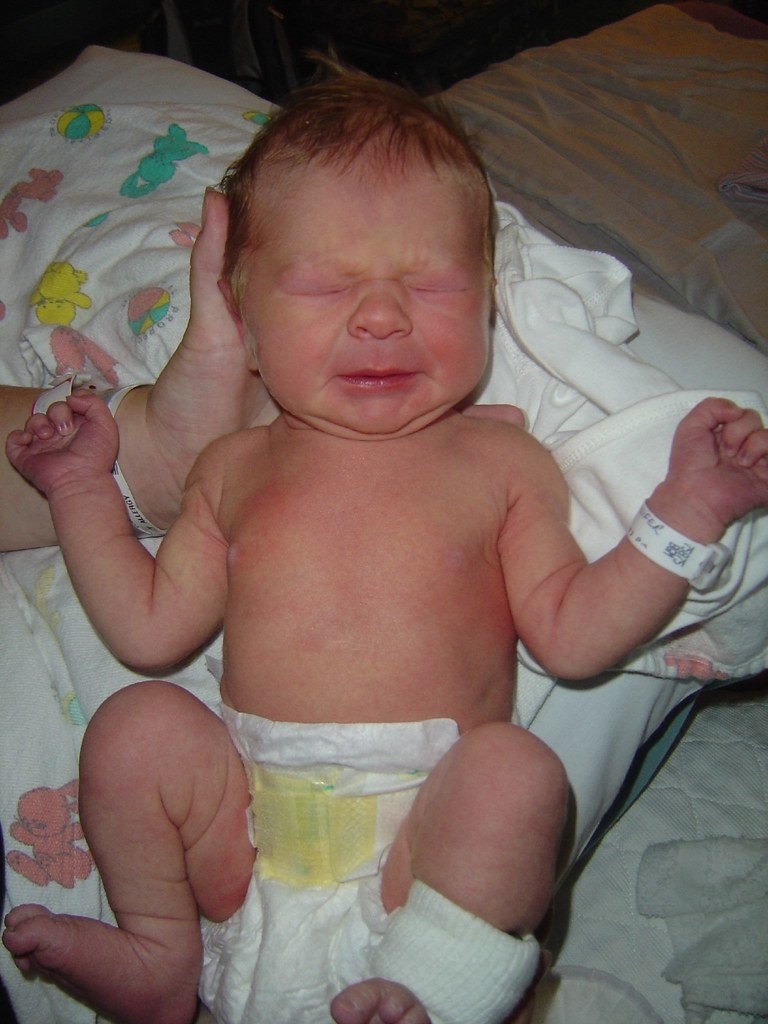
[[625, 500, 731, 592], [31, 362, 169, 541]]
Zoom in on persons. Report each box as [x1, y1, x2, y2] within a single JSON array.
[[1, 78, 767, 1024]]
